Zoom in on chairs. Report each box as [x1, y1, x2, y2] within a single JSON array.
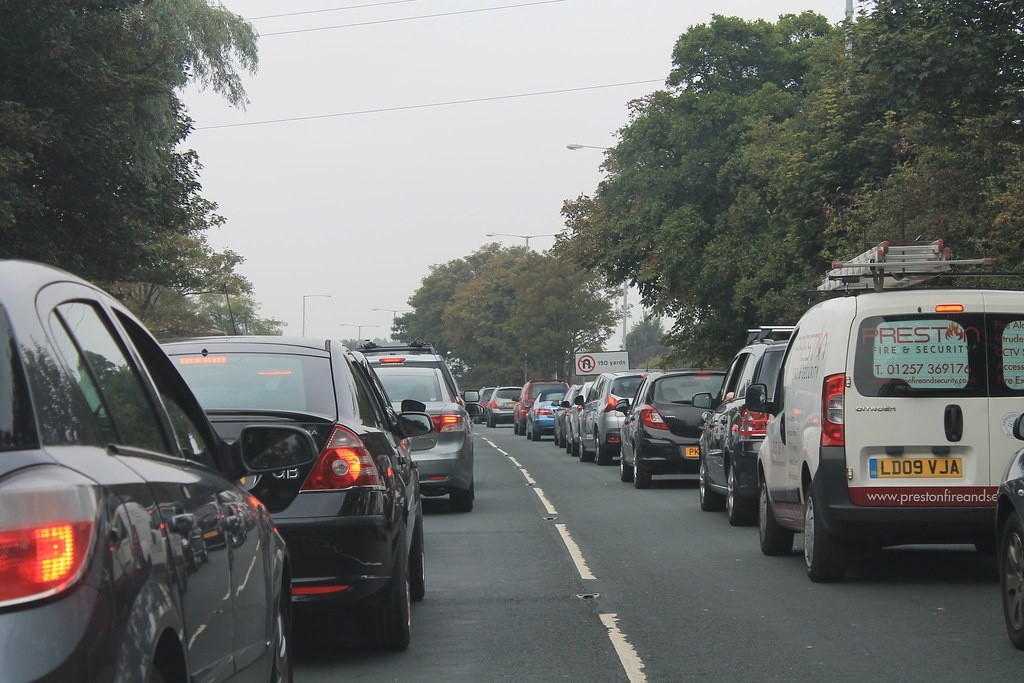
[[406, 384, 431, 401], [626, 382, 638, 394], [660, 387, 681, 401], [614, 384, 625, 393]]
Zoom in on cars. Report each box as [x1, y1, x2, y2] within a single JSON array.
[[994, 413, 1024, 654], [0, 257, 319, 682], [150, 333, 438, 652], [559, 381, 596, 459], [551, 384, 584, 449], [617, 368, 732, 491], [574, 370, 661, 466], [523, 389, 569, 441]]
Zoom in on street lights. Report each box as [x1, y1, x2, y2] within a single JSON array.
[[373, 308, 416, 336], [302, 294, 332, 336], [485, 231, 565, 255], [340, 323, 381, 341]]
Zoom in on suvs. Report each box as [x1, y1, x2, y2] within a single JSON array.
[[476, 385, 500, 424], [347, 336, 481, 512], [510, 380, 570, 436], [691, 324, 797, 525], [486, 386, 522, 428]]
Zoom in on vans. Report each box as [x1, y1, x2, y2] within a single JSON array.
[[745, 240, 1024, 582]]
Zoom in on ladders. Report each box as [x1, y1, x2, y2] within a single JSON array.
[[813, 238, 953, 292]]
